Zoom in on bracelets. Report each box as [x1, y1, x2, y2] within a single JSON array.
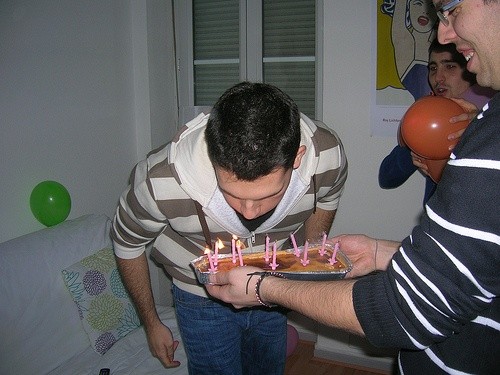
[[246, 271, 288, 309]]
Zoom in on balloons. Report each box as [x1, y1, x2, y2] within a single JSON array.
[[286, 325, 299, 358], [30, 181, 71, 227], [401, 96, 470, 159]]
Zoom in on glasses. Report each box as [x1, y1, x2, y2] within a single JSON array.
[[436, 0, 464, 27]]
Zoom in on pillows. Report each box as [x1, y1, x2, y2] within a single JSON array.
[[60, 243, 144, 358]]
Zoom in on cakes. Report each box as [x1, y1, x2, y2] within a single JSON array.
[[196, 246, 347, 272]]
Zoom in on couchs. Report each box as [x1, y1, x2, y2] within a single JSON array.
[[0, 214, 188, 375]]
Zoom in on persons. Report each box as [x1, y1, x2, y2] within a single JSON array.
[[378, 36, 497, 211], [205, 0, 500, 375], [110, 80, 348, 375]]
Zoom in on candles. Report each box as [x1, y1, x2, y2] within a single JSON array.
[[205, 231, 341, 272]]
